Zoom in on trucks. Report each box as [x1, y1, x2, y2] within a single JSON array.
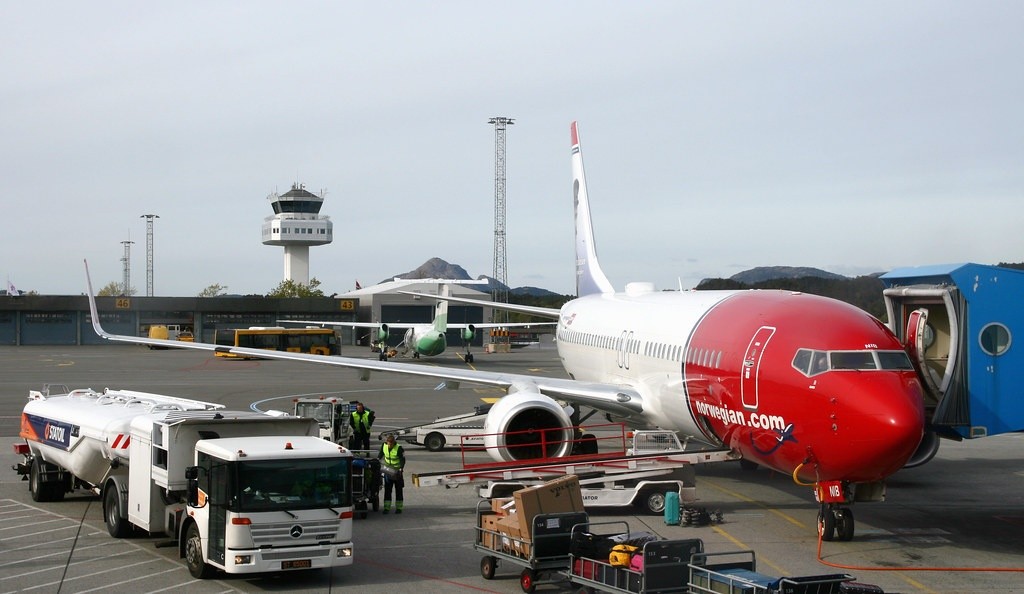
[[146, 325, 168, 350]]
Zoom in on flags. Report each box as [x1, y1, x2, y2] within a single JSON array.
[[8, 281, 20, 296]]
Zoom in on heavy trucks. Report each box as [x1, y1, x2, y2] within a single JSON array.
[[11, 387, 356, 580]]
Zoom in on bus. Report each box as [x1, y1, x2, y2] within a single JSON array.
[[213, 326, 342, 361]]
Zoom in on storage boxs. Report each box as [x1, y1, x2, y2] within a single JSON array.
[[693, 568, 779, 594], [481, 473, 586, 561]]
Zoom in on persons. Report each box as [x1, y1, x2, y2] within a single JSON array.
[[349, 403, 375, 457], [377, 435, 406, 514]]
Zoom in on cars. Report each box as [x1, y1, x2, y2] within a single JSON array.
[[165, 325, 180, 339]]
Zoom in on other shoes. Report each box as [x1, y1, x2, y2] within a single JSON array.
[[366, 452, 370, 457], [383, 509, 390, 515], [394, 508, 402, 515]]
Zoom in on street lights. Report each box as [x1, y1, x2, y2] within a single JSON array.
[[141, 214, 159, 297], [121, 241, 134, 295], [489, 116, 514, 353]]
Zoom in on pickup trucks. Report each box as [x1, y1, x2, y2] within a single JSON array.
[[175, 330, 195, 342]]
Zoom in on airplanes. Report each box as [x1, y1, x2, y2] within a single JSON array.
[[84, 120, 937, 544], [276, 284, 559, 363]]
